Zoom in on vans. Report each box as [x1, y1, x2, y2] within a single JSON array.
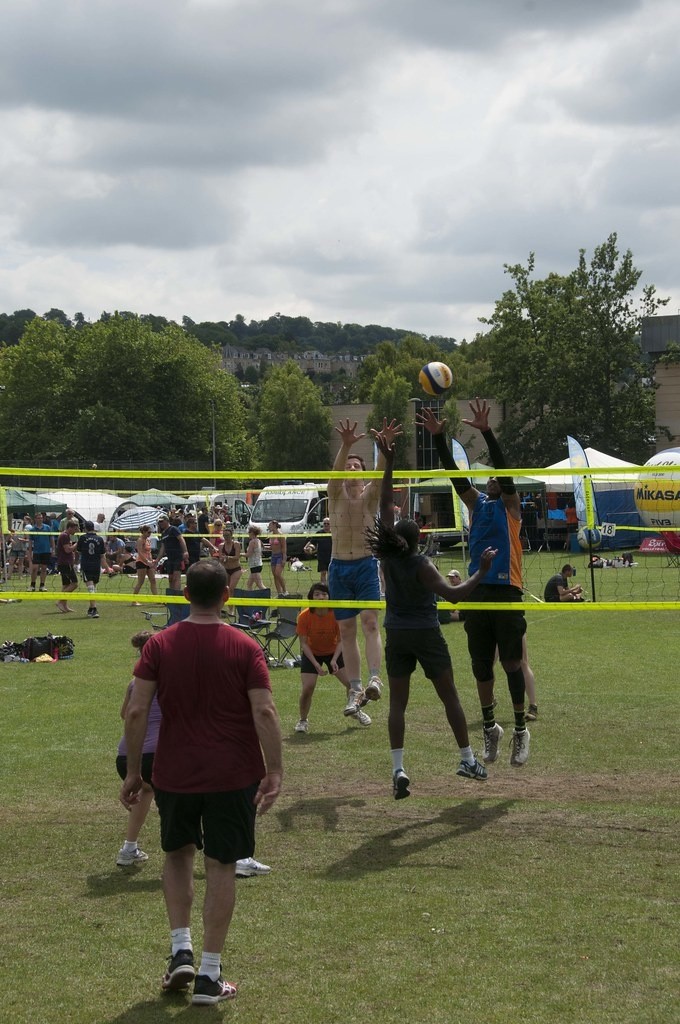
[[231, 481, 333, 559], [180, 492, 253, 519]]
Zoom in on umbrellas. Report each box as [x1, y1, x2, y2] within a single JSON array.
[[109, 506, 166, 531]]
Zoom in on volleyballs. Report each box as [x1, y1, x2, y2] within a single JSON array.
[[417, 361, 453, 396]]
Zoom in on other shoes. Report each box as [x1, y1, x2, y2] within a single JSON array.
[[27, 586, 35, 591], [55, 603, 77, 612], [39, 587, 48, 592]]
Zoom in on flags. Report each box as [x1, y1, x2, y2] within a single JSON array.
[[567, 435, 598, 528], [452, 439, 471, 528]]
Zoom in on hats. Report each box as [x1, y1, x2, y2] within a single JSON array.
[[157, 514, 170, 522], [446, 569, 460, 577]]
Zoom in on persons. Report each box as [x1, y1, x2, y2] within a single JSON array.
[[240, 525, 266, 591], [304, 517, 333, 585], [443, 570, 467, 621], [565, 501, 578, 533], [544, 564, 585, 602], [116, 633, 272, 876], [588, 552, 638, 568], [415, 397, 538, 766], [294, 583, 371, 732], [364, 434, 500, 799], [268, 521, 289, 598], [119, 556, 285, 1005], [328, 416, 405, 715], [0, 504, 243, 618]]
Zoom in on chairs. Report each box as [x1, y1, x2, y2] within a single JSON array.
[[142, 589, 305, 666]]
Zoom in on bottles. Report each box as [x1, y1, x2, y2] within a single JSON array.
[[249, 611, 262, 624]]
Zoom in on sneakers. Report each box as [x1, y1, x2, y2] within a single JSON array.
[[525, 704, 538, 721], [508, 727, 531, 767], [391, 768, 411, 800], [481, 723, 504, 764], [236, 857, 271, 876], [87, 607, 99, 618], [456, 752, 489, 780], [344, 687, 369, 716], [191, 963, 238, 1005], [116, 848, 149, 865], [161, 949, 196, 989], [365, 675, 384, 700], [295, 718, 309, 733], [351, 710, 372, 726]]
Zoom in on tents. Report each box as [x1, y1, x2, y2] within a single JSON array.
[[525, 448, 661, 551], [128, 487, 196, 509], [4, 489, 67, 514], [408, 461, 550, 552]]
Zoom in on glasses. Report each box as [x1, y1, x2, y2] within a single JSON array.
[[148, 532, 151, 533]]
